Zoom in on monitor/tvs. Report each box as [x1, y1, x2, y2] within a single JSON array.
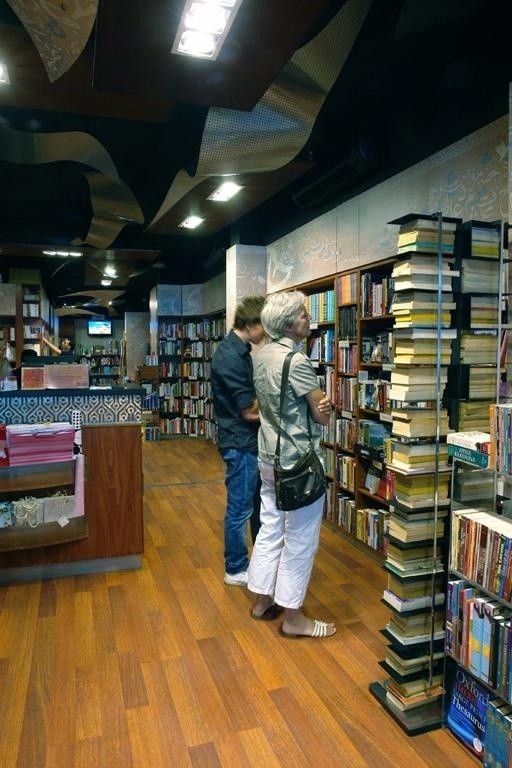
[[87, 320, 112, 335]]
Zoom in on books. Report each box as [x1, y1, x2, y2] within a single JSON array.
[[303, 274, 394, 558], [140, 318, 226, 444], [81, 342, 121, 386], [381, 221, 512, 712], [447, 669, 511, 768], [4, 290, 59, 361]]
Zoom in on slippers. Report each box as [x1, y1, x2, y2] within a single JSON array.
[[281, 619, 336, 638], [253, 594, 277, 618]]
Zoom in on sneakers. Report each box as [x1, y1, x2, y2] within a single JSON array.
[[224, 572, 248, 586]]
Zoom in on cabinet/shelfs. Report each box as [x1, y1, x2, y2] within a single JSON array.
[[0, 458, 89, 553], [156, 250, 398, 566], [136, 365, 160, 427], [79, 354, 120, 386], [15, 283, 43, 368], [440, 454, 512, 767], [0, 314, 56, 376], [369, 211, 511, 736]]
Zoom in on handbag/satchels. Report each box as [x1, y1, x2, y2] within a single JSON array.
[[273, 446, 328, 511]]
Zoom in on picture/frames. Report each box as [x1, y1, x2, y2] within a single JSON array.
[[20, 367, 46, 391]]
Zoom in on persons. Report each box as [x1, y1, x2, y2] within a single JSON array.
[[247, 292, 336, 638], [37, 331, 75, 356], [211, 295, 267, 586]]
